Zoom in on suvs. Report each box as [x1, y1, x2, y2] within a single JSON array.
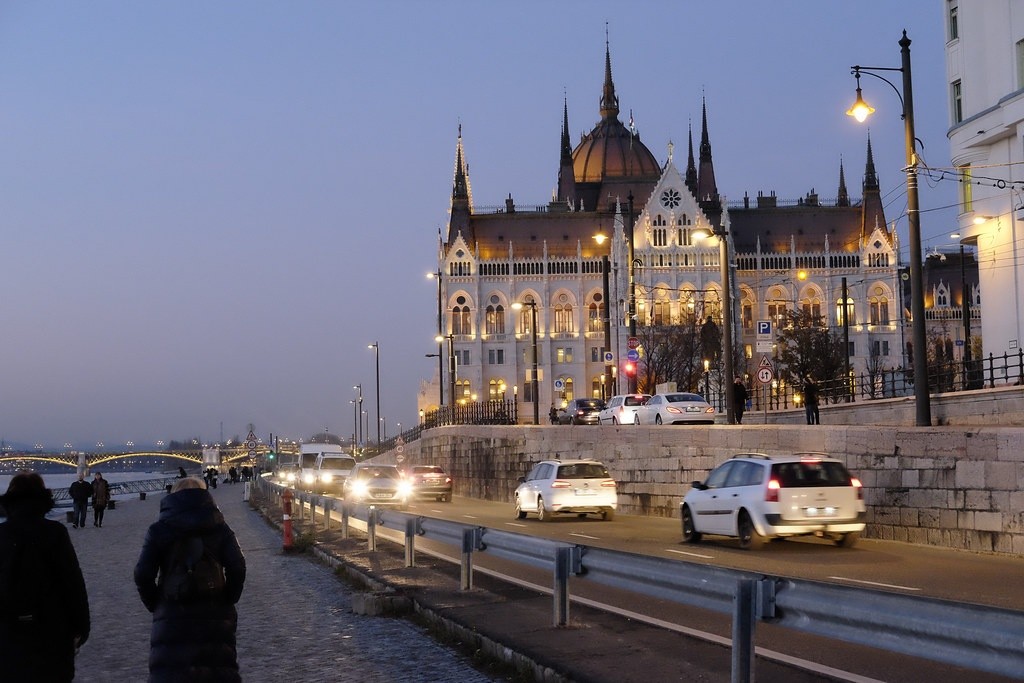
[[312, 451, 356, 495], [680, 451, 867, 551]]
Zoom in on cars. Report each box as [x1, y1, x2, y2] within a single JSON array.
[[344, 461, 453, 510], [560, 392, 715, 425], [277, 462, 299, 487], [512, 458, 618, 520]]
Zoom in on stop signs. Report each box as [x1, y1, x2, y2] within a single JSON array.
[[626, 337, 640, 350]]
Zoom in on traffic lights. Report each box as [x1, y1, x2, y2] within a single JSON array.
[[626, 362, 636, 378], [269, 450, 274, 460]]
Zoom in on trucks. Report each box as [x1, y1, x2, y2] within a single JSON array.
[[298, 444, 343, 491]]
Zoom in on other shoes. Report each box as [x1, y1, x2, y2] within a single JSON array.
[[93, 522, 98, 527], [73, 524, 78, 529], [99, 524, 102, 527]]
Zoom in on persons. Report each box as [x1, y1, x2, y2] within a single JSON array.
[[90, 472, 110, 527], [135, 477, 246, 683], [803, 378, 820, 425], [229, 467, 237, 484], [242, 465, 263, 482], [179, 467, 187, 478], [69, 474, 91, 528], [203, 468, 218, 489], [0, 473, 90, 683], [734, 376, 747, 424], [550, 403, 556, 424]]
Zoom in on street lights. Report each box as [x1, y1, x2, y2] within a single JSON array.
[[426, 267, 455, 425], [510, 299, 539, 425], [600, 373, 606, 401], [691, 225, 735, 426], [348, 383, 369, 445], [367, 341, 381, 445], [591, 190, 638, 394], [420, 409, 423, 425], [703, 359, 710, 405], [612, 367, 617, 397], [380, 416, 385, 442], [845, 29, 933, 426]]
